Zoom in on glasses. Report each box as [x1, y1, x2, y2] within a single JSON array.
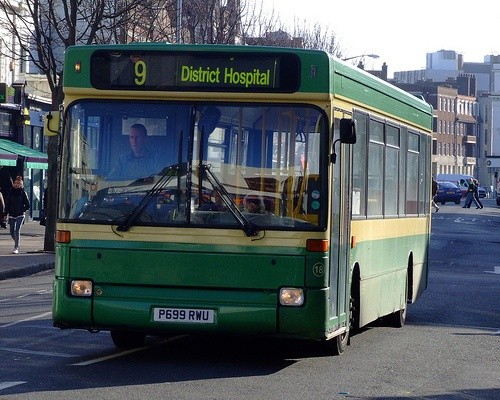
[[246, 198, 261, 205]]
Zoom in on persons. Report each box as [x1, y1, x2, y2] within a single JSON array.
[[163, 188, 275, 229], [432, 176, 439, 212], [0, 176, 48, 254], [461, 178, 483, 210], [105, 124, 163, 181]]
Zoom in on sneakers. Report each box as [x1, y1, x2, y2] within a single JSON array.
[[13, 250, 18, 254]]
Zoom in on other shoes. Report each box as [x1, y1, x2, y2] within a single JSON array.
[[436, 209, 439, 212], [462, 205, 483, 209]]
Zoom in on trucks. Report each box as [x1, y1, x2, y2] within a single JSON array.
[[438, 173, 487, 198]]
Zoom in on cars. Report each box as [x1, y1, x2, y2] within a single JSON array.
[[434, 181, 463, 205]]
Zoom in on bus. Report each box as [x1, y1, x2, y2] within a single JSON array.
[[43, 42, 435, 357]]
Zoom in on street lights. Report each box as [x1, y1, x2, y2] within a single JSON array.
[[342, 53, 381, 62]]
